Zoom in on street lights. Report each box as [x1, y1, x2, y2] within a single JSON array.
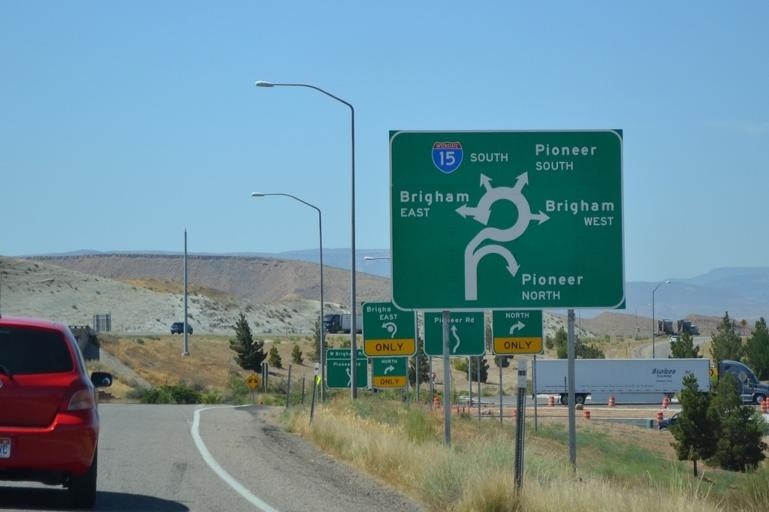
[[651, 279, 674, 360], [252, 79, 360, 401], [364, 254, 393, 263], [251, 191, 327, 407]]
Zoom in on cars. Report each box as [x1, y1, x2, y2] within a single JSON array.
[[669, 336, 680, 343], [1, 314, 117, 500]]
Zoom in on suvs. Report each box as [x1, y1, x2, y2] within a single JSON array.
[[171, 322, 194, 335]]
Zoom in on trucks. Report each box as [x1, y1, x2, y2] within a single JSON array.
[[690, 324, 700, 336], [530, 357, 769, 409], [323, 311, 363, 334]]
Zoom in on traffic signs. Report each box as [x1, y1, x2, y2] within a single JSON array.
[[492, 310, 546, 356], [326, 348, 368, 388], [388, 128, 631, 313], [423, 308, 488, 356], [370, 356, 408, 388], [362, 301, 419, 357]]
